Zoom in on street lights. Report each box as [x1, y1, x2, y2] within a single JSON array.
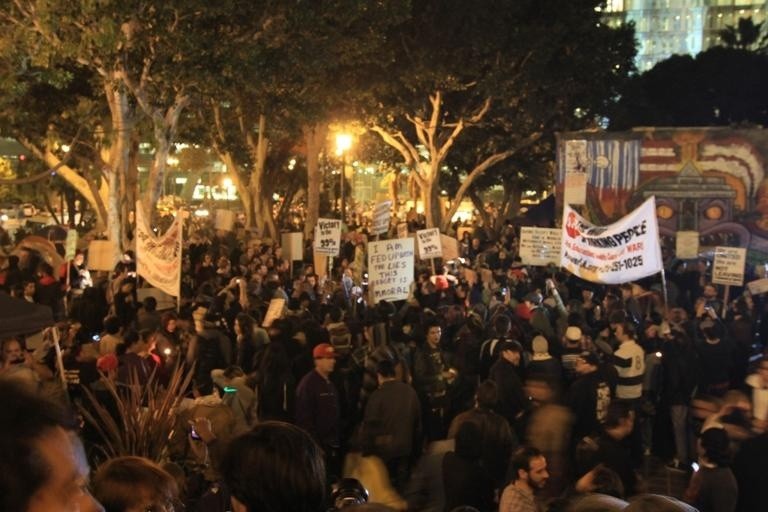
[[324, 125, 356, 223]]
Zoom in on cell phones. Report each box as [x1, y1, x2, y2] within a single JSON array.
[[690, 462, 700, 472], [191, 426, 200, 440], [92, 334, 101, 341]]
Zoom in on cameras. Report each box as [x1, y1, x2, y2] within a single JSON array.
[[330, 478, 368, 509]]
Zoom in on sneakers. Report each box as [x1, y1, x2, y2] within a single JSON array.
[[664, 458, 689, 474]]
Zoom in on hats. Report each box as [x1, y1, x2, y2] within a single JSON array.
[[533, 335, 548, 353], [521, 293, 544, 304], [429, 274, 448, 290], [500, 339, 524, 352], [96, 353, 119, 371], [311, 343, 341, 358], [566, 327, 582, 341]]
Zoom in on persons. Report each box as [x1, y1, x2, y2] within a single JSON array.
[[1, 191, 768, 510]]
